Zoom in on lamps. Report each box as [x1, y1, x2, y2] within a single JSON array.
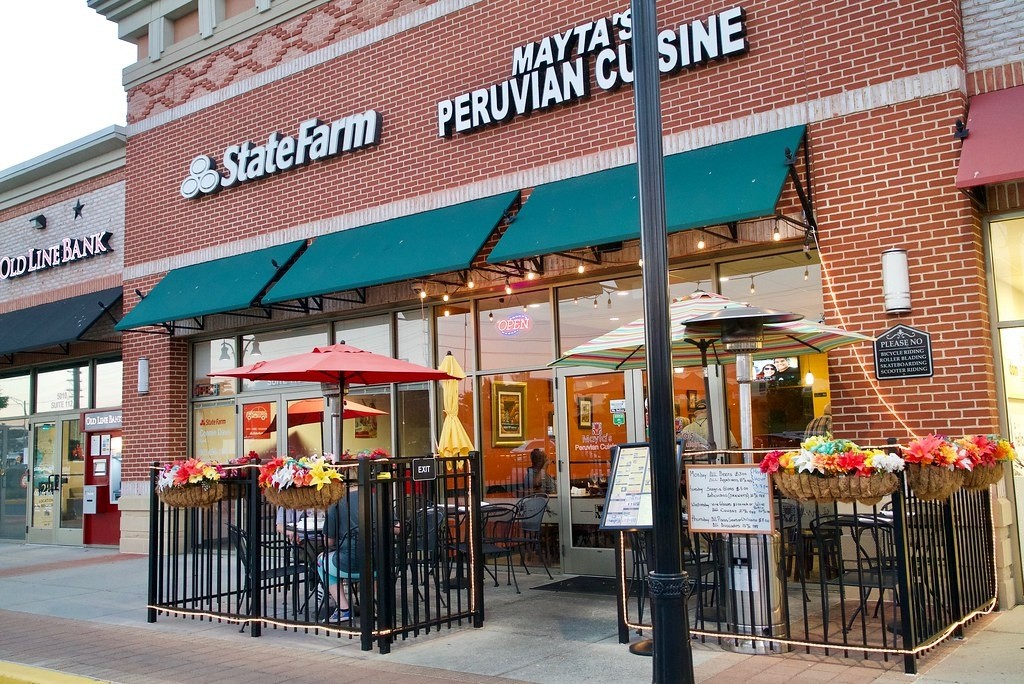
[[220, 339, 235, 360], [29, 215, 46, 229], [881, 248, 911, 313], [243, 338, 261, 356], [137, 358, 148, 394]]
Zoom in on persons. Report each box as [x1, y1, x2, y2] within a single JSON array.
[[523, 447, 582, 560], [679, 400, 738, 465], [752, 356, 800, 387], [275, 466, 400, 622]]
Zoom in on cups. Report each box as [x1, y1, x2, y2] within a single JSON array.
[[600, 474, 606, 483]]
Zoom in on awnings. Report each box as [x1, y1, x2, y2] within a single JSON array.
[[262, 189, 519, 305], [956, 85, 1024, 211], [114, 240, 307, 330], [0, 285, 124, 355], [486, 123, 806, 263]]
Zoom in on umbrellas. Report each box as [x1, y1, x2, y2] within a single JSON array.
[[207, 340, 462, 474], [436, 350, 474, 472], [546, 290, 876, 605], [260, 398, 389, 457]]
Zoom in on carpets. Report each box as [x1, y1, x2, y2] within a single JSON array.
[[529, 576, 714, 599]]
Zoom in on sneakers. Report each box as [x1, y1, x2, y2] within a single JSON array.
[[322, 608, 354, 623]]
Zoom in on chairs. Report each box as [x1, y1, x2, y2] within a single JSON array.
[[784, 496, 947, 655], [627, 523, 718, 638], [226, 495, 554, 633]]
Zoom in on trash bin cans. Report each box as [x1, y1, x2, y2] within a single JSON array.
[[720, 530, 796, 654]]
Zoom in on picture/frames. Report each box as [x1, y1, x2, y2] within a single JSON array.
[[578, 397, 593, 429], [687, 390, 697, 411], [491, 381, 527, 448]]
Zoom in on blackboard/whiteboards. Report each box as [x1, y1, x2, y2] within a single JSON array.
[[685, 463, 774, 534]]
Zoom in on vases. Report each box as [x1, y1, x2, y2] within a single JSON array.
[[772, 470, 899, 506], [263, 482, 345, 509], [906, 464, 968, 500], [158, 482, 223, 507], [963, 461, 1004, 489]]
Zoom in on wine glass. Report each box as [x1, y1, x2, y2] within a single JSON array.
[[591, 474, 598, 485]]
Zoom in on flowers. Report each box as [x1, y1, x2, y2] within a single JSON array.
[[154, 450, 345, 491], [759, 432, 1017, 478]]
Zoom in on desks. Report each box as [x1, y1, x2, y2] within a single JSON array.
[[825, 513, 943, 617]]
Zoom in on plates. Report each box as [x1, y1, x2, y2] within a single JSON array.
[[433, 504, 455, 508]]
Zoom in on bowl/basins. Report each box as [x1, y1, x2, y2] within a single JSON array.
[[301, 517, 325, 528]]
